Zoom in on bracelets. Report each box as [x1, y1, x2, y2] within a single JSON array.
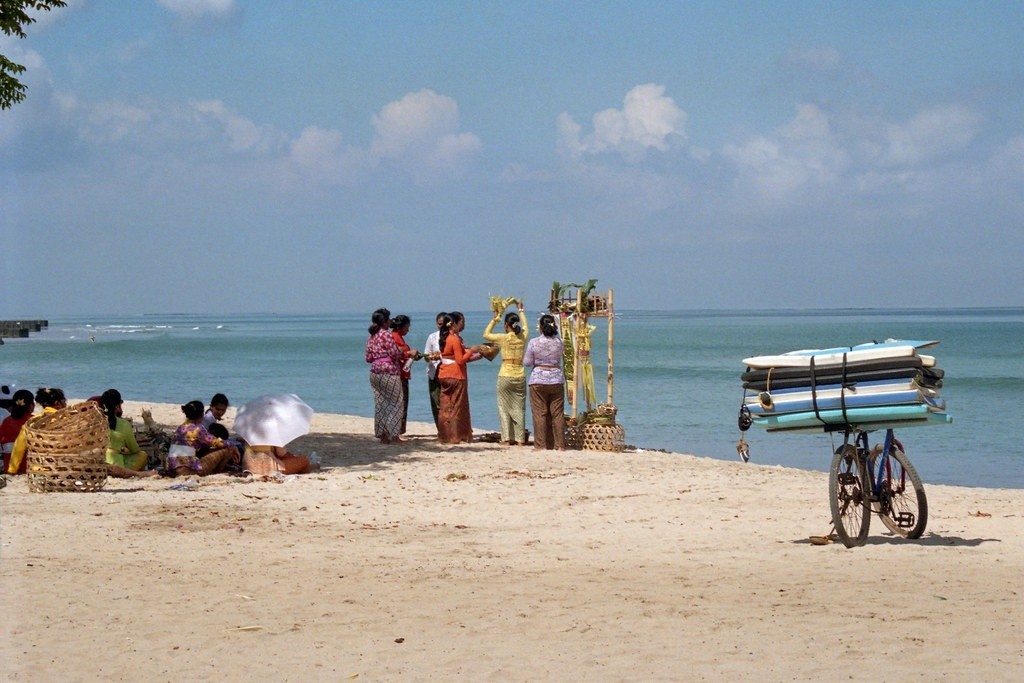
[[518, 309, 524, 312]]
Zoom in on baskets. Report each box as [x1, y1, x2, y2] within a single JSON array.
[[575, 422, 625, 453], [26, 397, 110, 493]]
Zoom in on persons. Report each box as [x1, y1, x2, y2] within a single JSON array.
[[196, 423, 229, 459], [483, 302, 529, 446], [168, 401, 240, 477], [0, 384, 16, 473], [6, 388, 158, 478], [438, 311, 481, 444], [365, 307, 404, 446], [523, 315, 566, 451], [241, 437, 320, 475], [202, 393, 244, 458], [424, 312, 448, 432], [0, 389, 35, 476], [390, 315, 418, 442], [99, 389, 148, 478]]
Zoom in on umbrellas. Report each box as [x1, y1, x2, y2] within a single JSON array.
[[231, 393, 314, 448]]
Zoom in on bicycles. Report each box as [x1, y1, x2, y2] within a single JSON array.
[[829, 426, 929, 546]]
